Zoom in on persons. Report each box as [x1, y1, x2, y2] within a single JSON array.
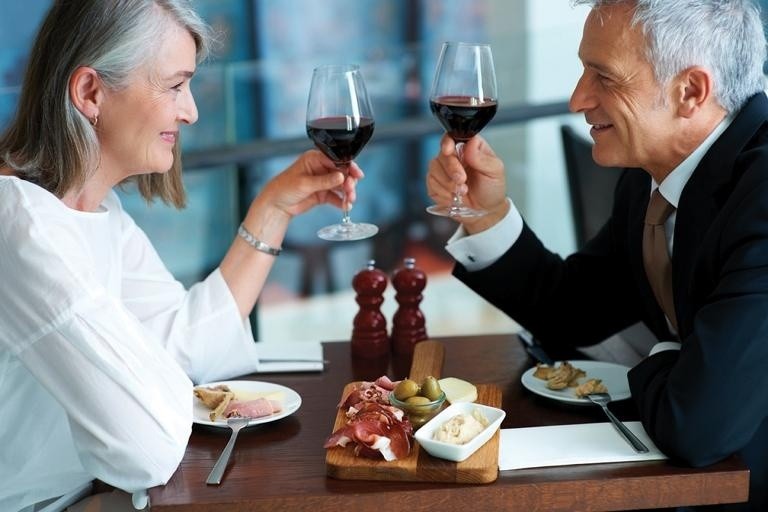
[[423, 1, 766, 512], [1, 0, 365, 512]]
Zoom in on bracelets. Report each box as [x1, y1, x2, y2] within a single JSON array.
[[237, 222, 282, 257]]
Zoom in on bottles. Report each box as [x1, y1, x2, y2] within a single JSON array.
[[390, 256, 432, 358], [348, 259, 393, 360]]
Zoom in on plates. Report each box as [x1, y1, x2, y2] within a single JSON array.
[[189, 381, 303, 429], [519, 359, 634, 407]]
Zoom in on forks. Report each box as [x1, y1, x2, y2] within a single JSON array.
[[205, 414, 252, 487], [581, 387, 647, 456]]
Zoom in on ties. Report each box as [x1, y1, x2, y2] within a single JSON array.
[[643, 188, 678, 327]]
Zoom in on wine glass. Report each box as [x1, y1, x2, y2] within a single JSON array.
[[423, 37, 499, 219], [304, 61, 379, 242]]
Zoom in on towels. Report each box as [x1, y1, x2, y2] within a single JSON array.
[[240, 334, 332, 372], [495, 417, 667, 474]]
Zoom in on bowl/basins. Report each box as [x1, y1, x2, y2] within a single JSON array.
[[386, 388, 446, 427], [413, 401, 506, 461]]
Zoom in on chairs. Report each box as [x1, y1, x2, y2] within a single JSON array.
[[558, 122, 620, 254]]
